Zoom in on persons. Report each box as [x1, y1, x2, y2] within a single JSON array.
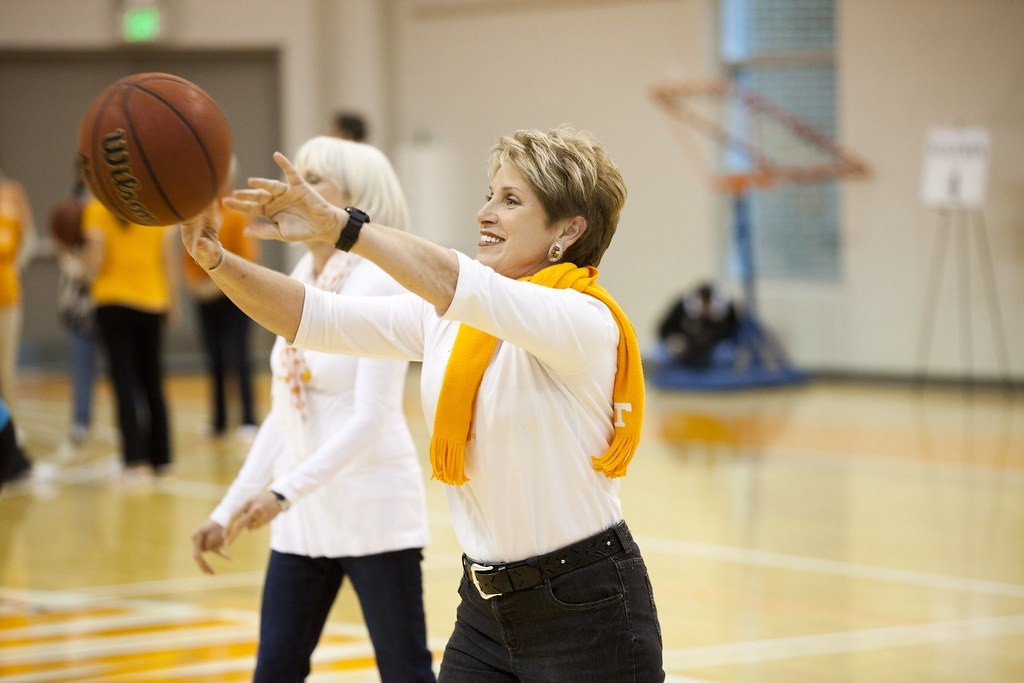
[[658, 284, 738, 369], [191, 133, 437, 683], [181, 127, 664, 683], [0, 111, 372, 474]]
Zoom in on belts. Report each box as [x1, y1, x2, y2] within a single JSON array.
[[461, 520, 632, 603]]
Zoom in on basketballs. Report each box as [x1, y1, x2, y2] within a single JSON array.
[[76, 71, 232, 226]]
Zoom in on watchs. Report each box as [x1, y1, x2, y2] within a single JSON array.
[[335, 206, 370, 253]]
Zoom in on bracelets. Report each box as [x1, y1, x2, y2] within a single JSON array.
[[194, 245, 225, 271], [272, 491, 289, 510]]
